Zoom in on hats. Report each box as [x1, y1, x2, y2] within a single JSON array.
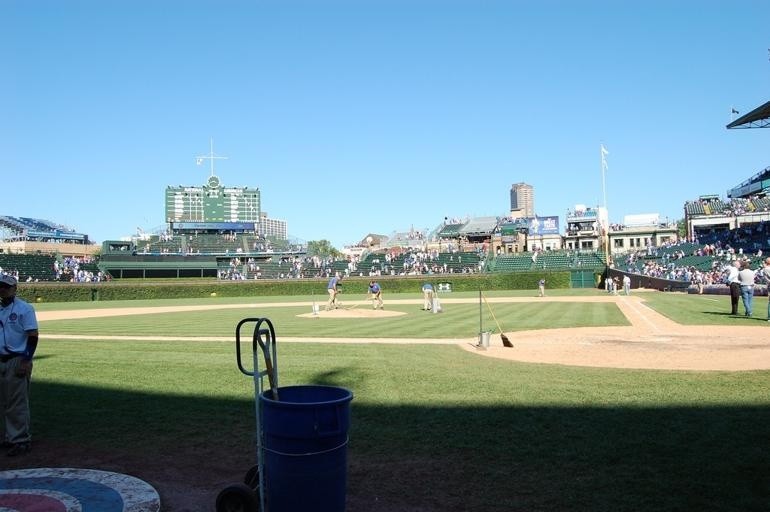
[[1, 274, 18, 288]]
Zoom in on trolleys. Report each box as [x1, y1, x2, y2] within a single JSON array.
[[216, 318, 279, 512]]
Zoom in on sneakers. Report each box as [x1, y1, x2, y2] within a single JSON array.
[[0, 438, 32, 457]]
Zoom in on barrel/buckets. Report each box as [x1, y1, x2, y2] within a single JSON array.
[[481, 330, 491, 348], [313, 304, 319, 313]]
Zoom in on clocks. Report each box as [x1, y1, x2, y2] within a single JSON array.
[[207, 175, 220, 189]]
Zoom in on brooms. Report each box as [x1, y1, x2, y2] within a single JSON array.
[[481, 292, 515, 348]]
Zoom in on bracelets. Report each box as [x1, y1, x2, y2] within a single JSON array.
[[23, 335, 38, 360]]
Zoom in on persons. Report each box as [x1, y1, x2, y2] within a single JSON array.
[[422, 283, 437, 312], [760, 257, 770, 320], [727, 261, 741, 315], [144, 232, 276, 254], [604, 194, 770, 299], [737, 262, 756, 316], [51, 254, 111, 282], [538, 278, 545, 297], [216, 253, 487, 280], [325, 275, 345, 310], [531, 250, 583, 269], [0, 267, 40, 283], [364, 229, 488, 252], [367, 280, 384, 309], [0, 275, 38, 459]]
